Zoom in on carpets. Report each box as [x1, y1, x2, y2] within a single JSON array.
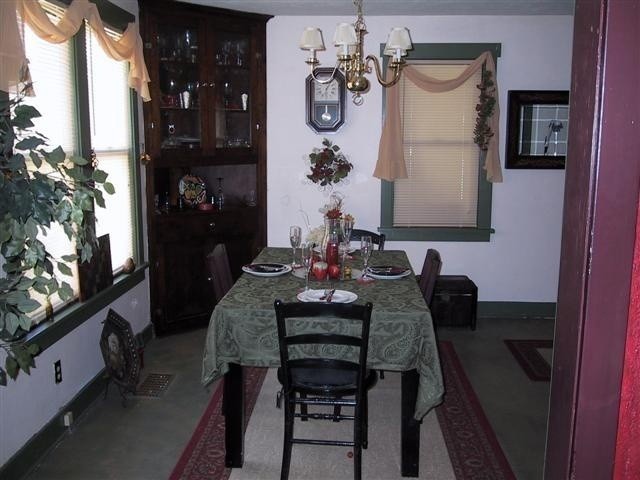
[[168, 339, 517, 480], [504, 339, 554, 382]]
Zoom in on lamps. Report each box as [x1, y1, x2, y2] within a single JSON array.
[[299, 0, 412, 106]]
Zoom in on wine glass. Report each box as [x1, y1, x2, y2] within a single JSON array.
[[290, 225, 313, 291], [341, 220, 353, 259], [360, 236, 372, 282], [217, 38, 249, 65]]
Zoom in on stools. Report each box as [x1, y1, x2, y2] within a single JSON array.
[[415, 275, 477, 331]]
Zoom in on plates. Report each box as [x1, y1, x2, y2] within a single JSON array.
[[242, 263, 293, 277], [296, 289, 359, 304], [363, 266, 411, 280], [177, 136, 201, 142]]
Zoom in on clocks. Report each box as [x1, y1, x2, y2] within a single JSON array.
[[306, 67, 347, 135]]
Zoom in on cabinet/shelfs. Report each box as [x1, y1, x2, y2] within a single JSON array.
[[138, 0, 275, 340]]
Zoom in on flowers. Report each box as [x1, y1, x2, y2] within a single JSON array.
[[306, 138, 353, 218]]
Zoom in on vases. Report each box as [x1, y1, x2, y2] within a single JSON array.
[[321, 215, 349, 264]]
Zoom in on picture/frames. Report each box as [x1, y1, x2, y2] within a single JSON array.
[[505, 89, 570, 170]]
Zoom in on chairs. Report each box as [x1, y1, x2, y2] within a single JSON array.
[[207, 243, 234, 303], [308, 229, 386, 251], [273, 299, 373, 480], [380, 249, 442, 379]]
